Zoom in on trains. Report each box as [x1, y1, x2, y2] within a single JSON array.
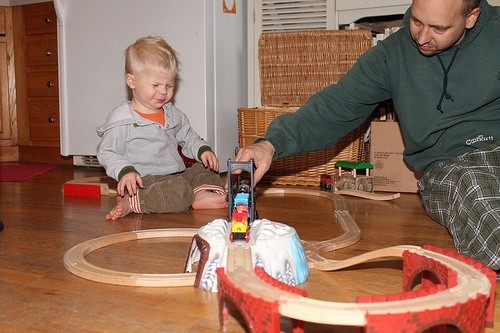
[[230, 179, 250, 243]]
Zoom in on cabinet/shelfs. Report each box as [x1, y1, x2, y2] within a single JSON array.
[[0, 0, 71, 165]]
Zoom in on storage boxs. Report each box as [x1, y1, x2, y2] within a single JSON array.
[[237, 29, 373, 186], [370, 122, 418, 192]]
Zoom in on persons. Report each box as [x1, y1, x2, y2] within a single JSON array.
[[95, 35, 230, 220], [231, 0, 500, 274]]
